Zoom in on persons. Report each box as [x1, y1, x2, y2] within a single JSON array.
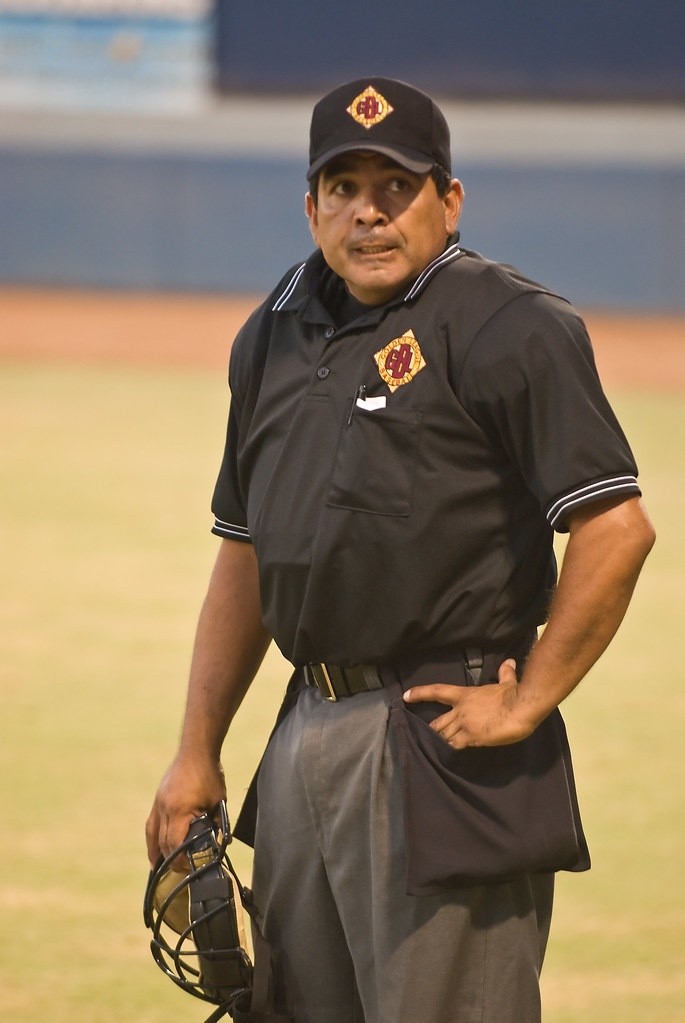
[[143, 77, 657, 1023]]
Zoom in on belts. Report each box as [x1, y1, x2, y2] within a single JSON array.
[[296, 659, 399, 703]]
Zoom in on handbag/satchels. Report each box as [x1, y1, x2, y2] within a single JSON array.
[[389, 655, 592, 887]]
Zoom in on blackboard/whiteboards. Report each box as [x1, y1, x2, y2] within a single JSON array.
[[209, 0, 685, 104]]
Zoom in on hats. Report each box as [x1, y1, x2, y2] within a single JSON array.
[[306, 78, 450, 180]]
